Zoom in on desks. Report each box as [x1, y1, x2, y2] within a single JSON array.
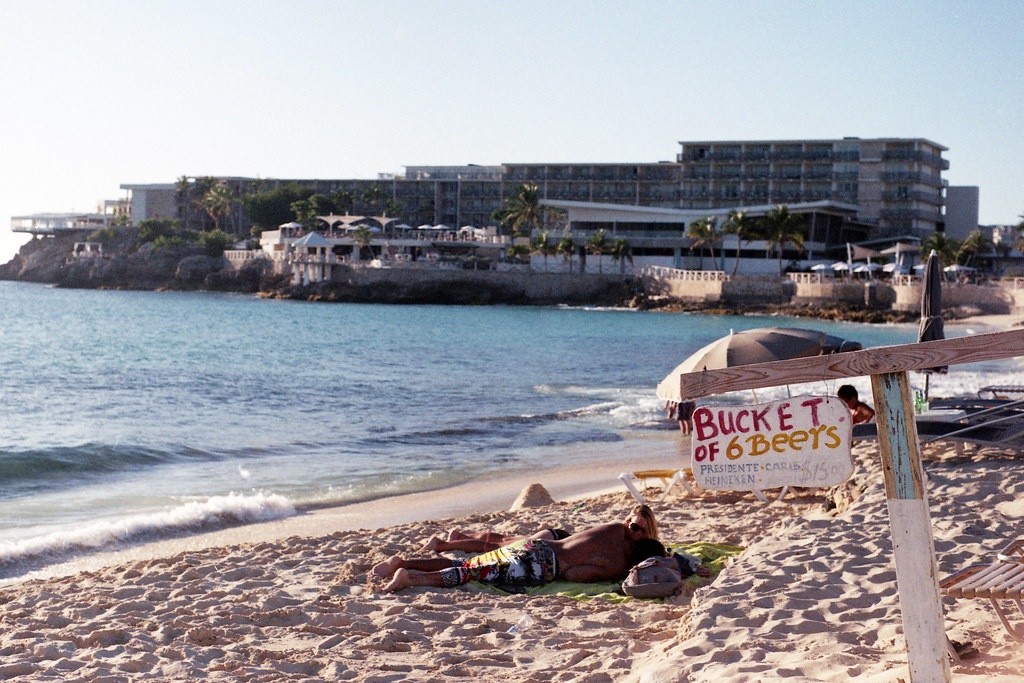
[[914, 410, 968, 423]]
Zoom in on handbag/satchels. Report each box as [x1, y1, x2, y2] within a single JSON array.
[[623, 554, 682, 599]]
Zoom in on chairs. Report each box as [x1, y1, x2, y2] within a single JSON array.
[[844, 384, 1024, 450]]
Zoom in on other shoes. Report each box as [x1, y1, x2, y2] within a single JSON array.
[[697, 565, 713, 577]]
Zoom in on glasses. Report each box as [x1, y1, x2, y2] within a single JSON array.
[[629, 521, 647, 533]]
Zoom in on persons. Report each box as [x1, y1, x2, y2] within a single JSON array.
[[663, 401, 696, 434], [418, 231, 474, 242], [837, 385, 875, 425], [373, 505, 713, 592], [956, 272, 985, 286]]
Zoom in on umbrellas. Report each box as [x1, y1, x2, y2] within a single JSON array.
[[292, 231, 332, 248], [279, 222, 302, 229], [656, 326, 862, 405], [811, 243, 925, 282], [348, 223, 450, 234], [913, 249, 949, 401], [943, 263, 976, 282]]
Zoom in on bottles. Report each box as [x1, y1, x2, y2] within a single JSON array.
[[914, 389, 929, 414]]
